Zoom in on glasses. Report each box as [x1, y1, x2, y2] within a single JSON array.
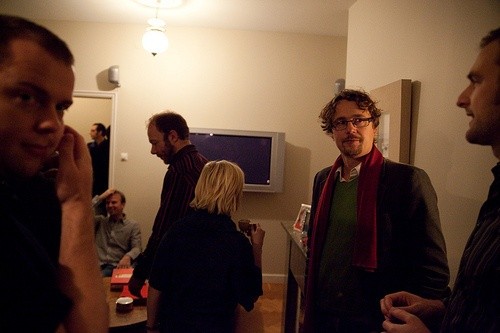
[[331, 117, 374, 131]]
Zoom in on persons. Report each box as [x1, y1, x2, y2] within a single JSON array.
[[92, 188, 142, 277], [380, 27, 500, 333], [306, 89, 450, 333], [146, 160, 266, 333], [0, 14, 111, 333], [87, 123, 110, 198], [128, 112, 209, 299]]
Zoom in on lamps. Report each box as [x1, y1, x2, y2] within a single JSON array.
[[137, 0, 182, 59]]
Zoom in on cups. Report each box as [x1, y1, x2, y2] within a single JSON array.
[[247, 223, 260, 236], [116, 297, 133, 313]]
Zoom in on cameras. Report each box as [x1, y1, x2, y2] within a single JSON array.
[[239, 220, 256, 236]]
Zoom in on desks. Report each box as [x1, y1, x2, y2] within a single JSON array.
[[280, 219, 312, 333], [101, 274, 147, 329]]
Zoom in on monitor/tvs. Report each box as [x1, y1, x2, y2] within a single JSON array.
[[189, 128, 285, 193]]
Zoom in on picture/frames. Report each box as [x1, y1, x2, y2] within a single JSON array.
[[293, 203, 312, 232]]
